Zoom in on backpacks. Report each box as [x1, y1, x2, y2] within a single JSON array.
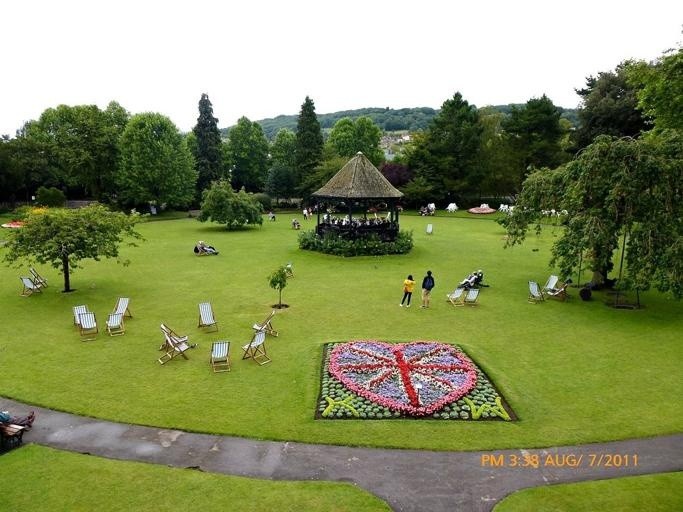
[[425, 279, 432, 289]]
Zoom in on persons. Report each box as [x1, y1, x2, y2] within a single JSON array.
[[421, 271, 435, 308], [420, 206, 428, 216], [459, 270, 484, 289], [0, 410, 35, 427], [268, 212, 276, 222], [290, 219, 301, 229], [303, 202, 322, 221], [400, 275, 416, 308]]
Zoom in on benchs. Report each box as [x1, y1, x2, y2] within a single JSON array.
[[65, 200, 98, 213]]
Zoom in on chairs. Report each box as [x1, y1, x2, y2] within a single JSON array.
[[241, 309, 279, 366], [198, 302, 220, 334], [155, 322, 196, 366], [0, 418, 25, 449], [72, 304, 98, 342], [426, 224, 433, 233], [527, 275, 568, 305], [446, 268, 489, 308], [104, 297, 133, 337], [19, 268, 48, 298], [209, 341, 232, 373]]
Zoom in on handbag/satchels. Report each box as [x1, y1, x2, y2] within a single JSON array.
[[403, 286, 409, 293]]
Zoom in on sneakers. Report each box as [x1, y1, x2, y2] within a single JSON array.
[[419, 305, 429, 309], [400, 304, 410, 308]]
